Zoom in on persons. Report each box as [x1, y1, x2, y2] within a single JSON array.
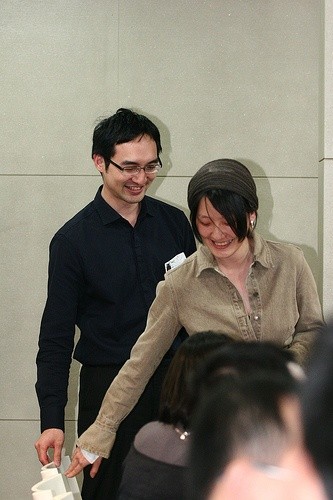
[[122, 322, 333, 500], [65, 158, 325, 500], [33, 107, 197, 500]]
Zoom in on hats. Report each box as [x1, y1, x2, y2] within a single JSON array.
[[187, 159, 259, 208]]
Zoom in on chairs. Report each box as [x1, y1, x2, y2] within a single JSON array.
[[53, 492, 74, 500], [40, 455, 83, 500], [31, 474, 66, 500]]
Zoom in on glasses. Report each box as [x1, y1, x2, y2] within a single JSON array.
[[104, 156, 162, 176]]
